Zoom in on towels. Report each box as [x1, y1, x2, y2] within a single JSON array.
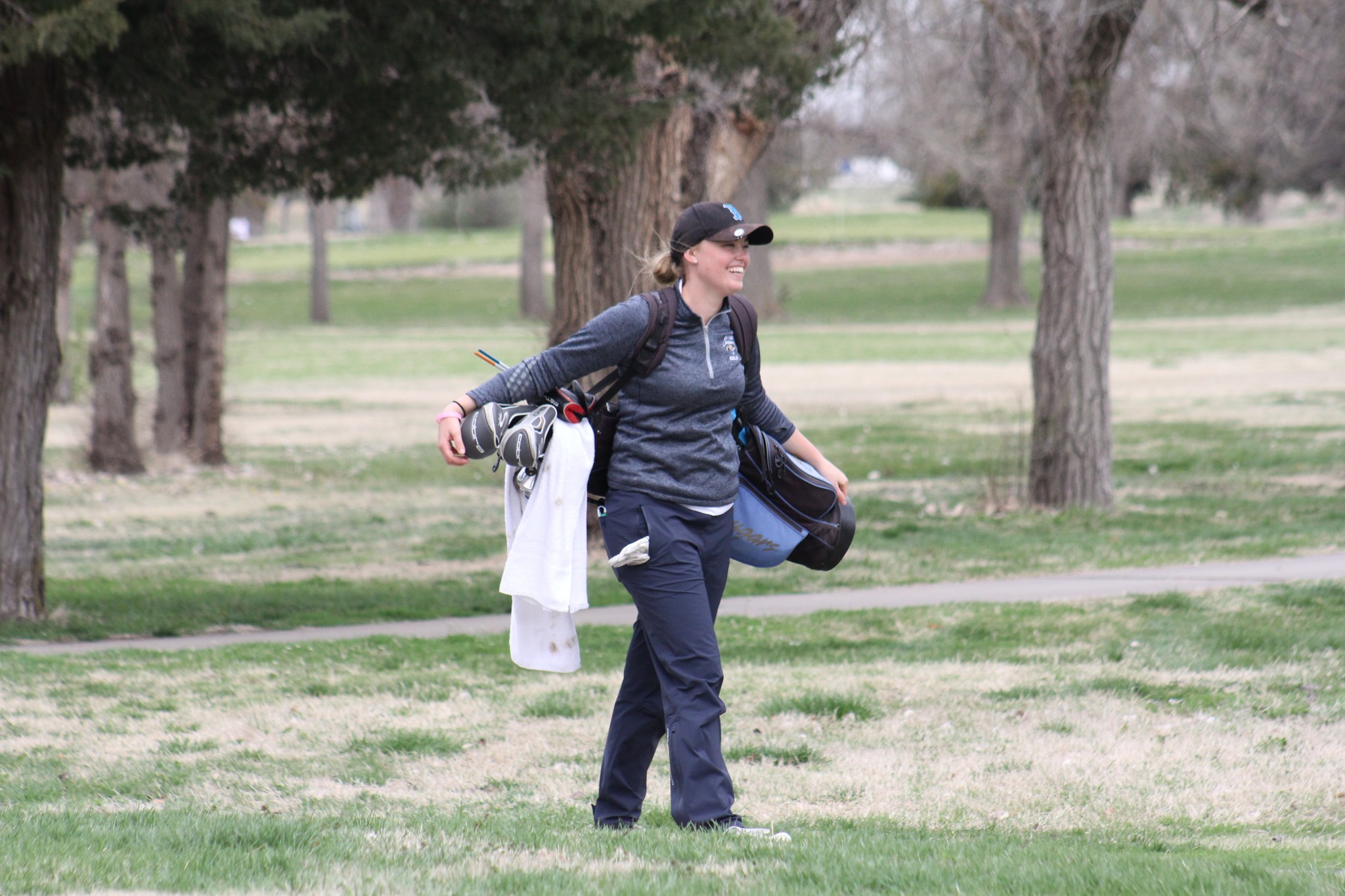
[[497, 415, 596, 674]]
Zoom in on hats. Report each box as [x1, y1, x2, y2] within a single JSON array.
[[671, 202, 773, 263]]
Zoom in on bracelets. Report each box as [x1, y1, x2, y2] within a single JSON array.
[[436, 413, 461, 421], [453, 400, 466, 418]]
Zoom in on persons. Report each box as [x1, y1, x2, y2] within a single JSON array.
[[436, 202, 849, 841]]
